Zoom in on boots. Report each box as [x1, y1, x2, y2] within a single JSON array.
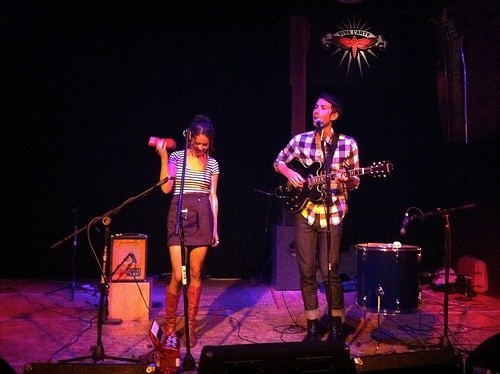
[[182, 284, 202, 348], [455, 277, 477, 297], [307, 318, 321, 343], [164, 286, 183, 348], [330, 316, 346, 344]]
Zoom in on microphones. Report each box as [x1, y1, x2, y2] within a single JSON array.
[[314, 117, 324, 129], [183, 128, 191, 137], [400, 212, 408, 234], [130, 252, 137, 263], [422, 272, 440, 281]]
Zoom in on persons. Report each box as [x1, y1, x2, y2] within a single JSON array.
[[273, 93, 359, 341], [157, 115, 220, 348]]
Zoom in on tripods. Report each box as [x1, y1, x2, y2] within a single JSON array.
[[44, 225, 95, 301], [406, 204, 475, 356], [173, 131, 198, 374], [51, 176, 176, 366]]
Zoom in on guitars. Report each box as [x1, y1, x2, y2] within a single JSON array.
[[272, 157, 393, 213]]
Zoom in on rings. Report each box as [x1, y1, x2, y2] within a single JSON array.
[[217, 241, 219, 243]]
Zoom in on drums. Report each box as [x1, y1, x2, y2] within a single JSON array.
[[355, 243, 421, 312]]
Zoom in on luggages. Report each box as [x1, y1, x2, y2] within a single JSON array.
[[457, 256, 488, 294]]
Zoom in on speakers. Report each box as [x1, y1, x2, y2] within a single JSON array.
[[198, 341, 356, 374], [271, 224, 302, 290]]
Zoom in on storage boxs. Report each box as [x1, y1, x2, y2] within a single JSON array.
[[105, 277, 152, 323], [110, 234, 149, 282]]
